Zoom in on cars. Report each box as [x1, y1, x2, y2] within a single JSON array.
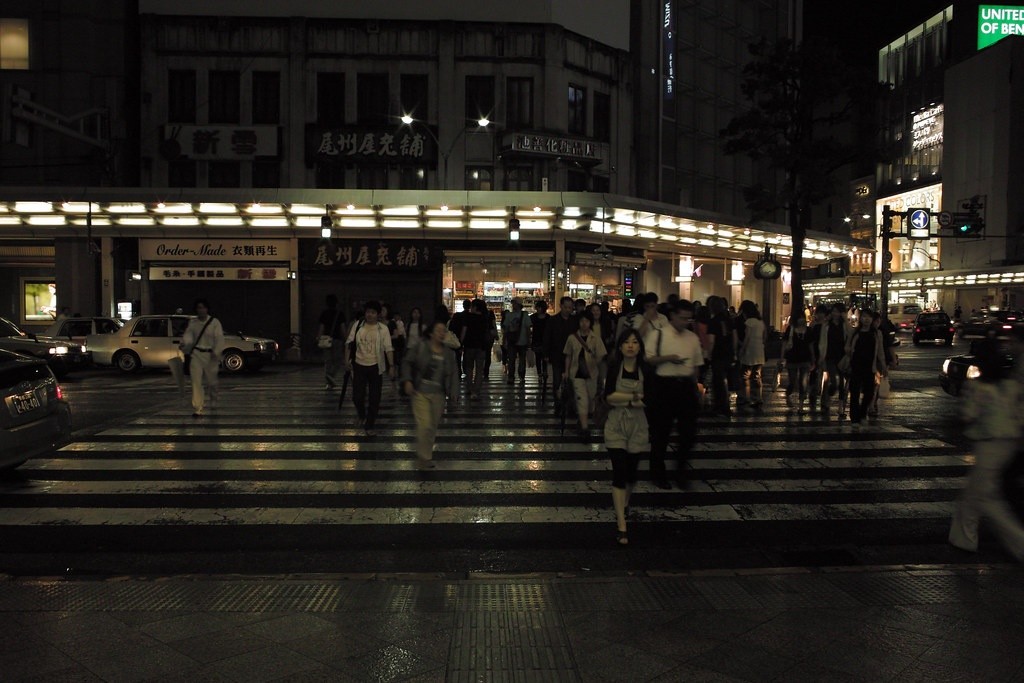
[[911, 312, 956, 346], [83, 314, 280, 377], [955, 308, 1024, 343], [34, 316, 142, 347], [938, 344, 1019, 398], [0, 346, 78, 473], [0, 316, 83, 381]]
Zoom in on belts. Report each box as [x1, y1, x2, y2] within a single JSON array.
[[193, 347, 213, 353]]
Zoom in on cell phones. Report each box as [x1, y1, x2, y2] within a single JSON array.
[[677, 357, 690, 362]]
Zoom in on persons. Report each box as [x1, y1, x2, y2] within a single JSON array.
[[181, 299, 227, 419], [316, 291, 1024, 562]]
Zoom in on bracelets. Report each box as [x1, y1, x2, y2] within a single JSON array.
[[629, 400, 632, 409], [390, 364, 394, 367]]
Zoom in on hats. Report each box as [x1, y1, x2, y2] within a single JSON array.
[[510, 297, 523, 304]]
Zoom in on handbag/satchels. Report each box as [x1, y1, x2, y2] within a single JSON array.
[[785, 324, 794, 351], [348, 320, 362, 364], [318, 336, 333, 347], [506, 311, 524, 344], [527, 349, 537, 368], [878, 378, 891, 399], [772, 364, 782, 393], [838, 355, 852, 374], [399, 377, 416, 401], [595, 394, 611, 427]]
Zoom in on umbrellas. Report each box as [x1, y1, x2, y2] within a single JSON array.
[[559, 375, 573, 436], [772, 360, 784, 395], [167, 346, 186, 399]]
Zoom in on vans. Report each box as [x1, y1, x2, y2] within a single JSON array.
[[887, 304, 923, 331]]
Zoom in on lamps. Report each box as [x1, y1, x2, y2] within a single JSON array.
[[127, 270, 144, 282], [506, 209, 522, 241], [318, 214, 338, 245], [286, 269, 298, 283]]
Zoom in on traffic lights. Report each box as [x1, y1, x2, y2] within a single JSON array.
[[828, 256, 850, 279], [753, 258, 782, 282], [953, 219, 983, 236]]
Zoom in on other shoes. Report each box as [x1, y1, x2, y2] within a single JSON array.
[[507, 378, 515, 384], [192, 407, 204, 417], [470, 393, 480, 401], [367, 428, 377, 439], [616, 530, 631, 546], [652, 479, 672, 490], [852, 422, 860, 428]]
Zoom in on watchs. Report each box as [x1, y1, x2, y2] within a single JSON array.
[[633, 391, 639, 402]]
